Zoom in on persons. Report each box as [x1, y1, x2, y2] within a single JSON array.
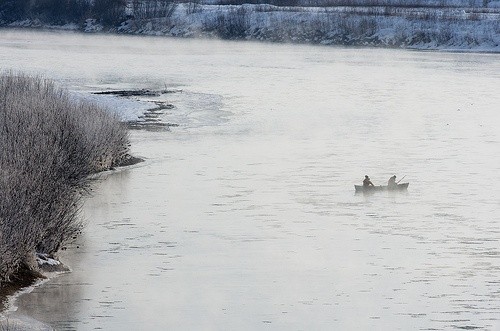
[[363, 176, 374, 188], [389, 176, 399, 188]]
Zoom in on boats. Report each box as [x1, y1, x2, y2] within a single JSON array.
[[354, 182, 409, 194]]
